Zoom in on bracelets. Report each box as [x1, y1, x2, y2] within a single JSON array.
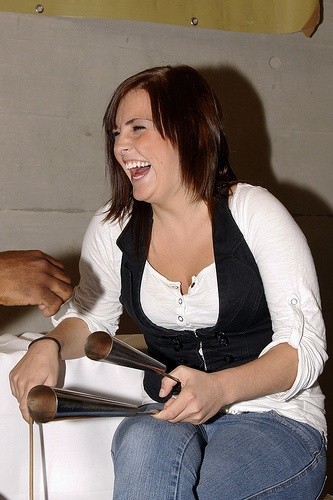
[[27, 336, 61, 353]]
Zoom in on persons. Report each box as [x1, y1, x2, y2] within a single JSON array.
[[0, 249, 75, 316], [9, 66, 333, 500]]
